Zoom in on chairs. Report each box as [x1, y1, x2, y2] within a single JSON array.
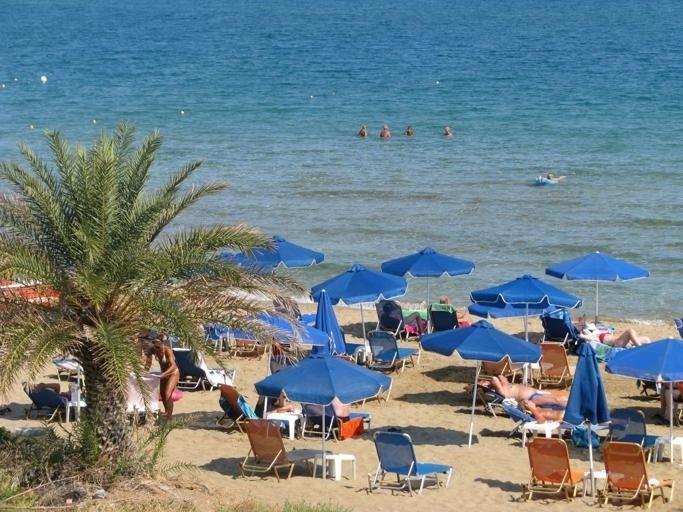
[[368, 431, 453, 497], [535, 341, 576, 391], [299, 400, 371, 442], [540, 307, 580, 351], [473, 360, 526, 384], [238, 418, 333, 482], [430, 306, 460, 331], [598, 441, 674, 509], [171, 346, 236, 392], [159, 309, 318, 361], [580, 326, 635, 361], [520, 437, 595, 501], [463, 379, 627, 441], [606, 405, 670, 465], [374, 300, 428, 343], [365, 329, 420, 373], [214, 383, 262, 433], [22, 382, 72, 424], [51, 355, 85, 384]]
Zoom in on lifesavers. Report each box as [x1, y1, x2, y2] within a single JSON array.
[[535, 176, 558, 184]]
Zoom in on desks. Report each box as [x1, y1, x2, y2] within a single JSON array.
[[583, 467, 606, 496], [314, 454, 357, 481]]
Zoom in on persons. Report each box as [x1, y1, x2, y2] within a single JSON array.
[[403, 126, 413, 136], [545, 173, 566, 182], [378, 124, 390, 137], [443, 127, 452, 136], [30, 380, 73, 402], [357, 125, 367, 137], [142, 340, 180, 426]]
